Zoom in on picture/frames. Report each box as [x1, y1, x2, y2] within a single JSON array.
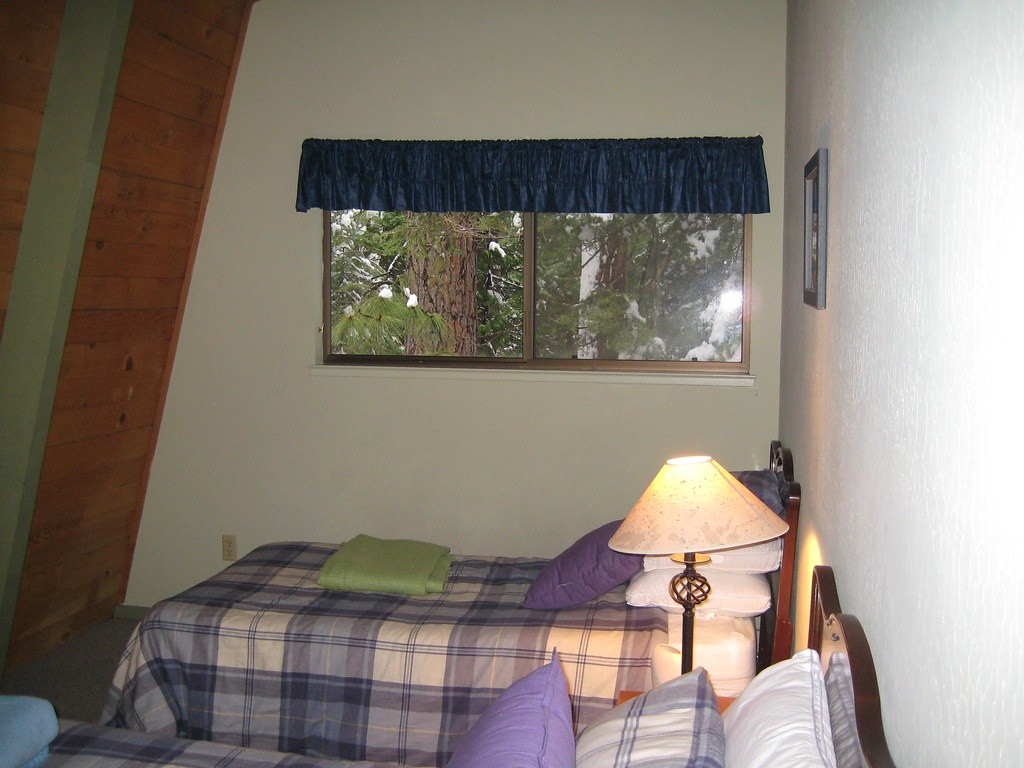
[[803, 148, 829, 310]]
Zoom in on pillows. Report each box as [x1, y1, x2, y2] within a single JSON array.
[[576, 667, 726, 768], [719, 648, 839, 768], [448, 648, 576, 768], [518, 518, 645, 610], [729, 469, 783, 515], [644, 538, 780, 574], [625, 568, 773, 617]]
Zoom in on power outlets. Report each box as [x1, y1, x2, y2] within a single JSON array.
[[222, 535, 237, 561]]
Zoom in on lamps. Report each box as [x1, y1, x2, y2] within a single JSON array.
[[608, 456, 790, 677]]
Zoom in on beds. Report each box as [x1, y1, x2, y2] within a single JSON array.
[[98, 440, 802, 768], [35, 566, 895, 768]]
